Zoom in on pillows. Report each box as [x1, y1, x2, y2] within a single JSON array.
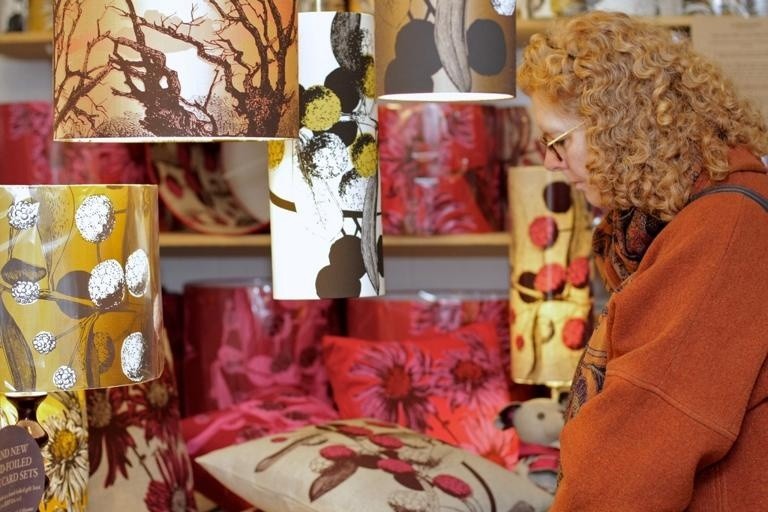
[[179, 386, 341, 512], [324, 320, 520, 470], [195, 417, 555, 512]]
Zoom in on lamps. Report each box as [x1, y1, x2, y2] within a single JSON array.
[[0, 183, 165, 512], [375, 0, 517, 104], [341, 284, 509, 342], [268, 12, 388, 340], [179, 276, 328, 402], [506, 167, 596, 404], [53, 0, 300, 141]]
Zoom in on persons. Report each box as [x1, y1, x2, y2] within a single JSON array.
[[516, 9, 764, 511]]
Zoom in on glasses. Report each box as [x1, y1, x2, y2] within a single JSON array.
[[539, 120, 586, 162]]
